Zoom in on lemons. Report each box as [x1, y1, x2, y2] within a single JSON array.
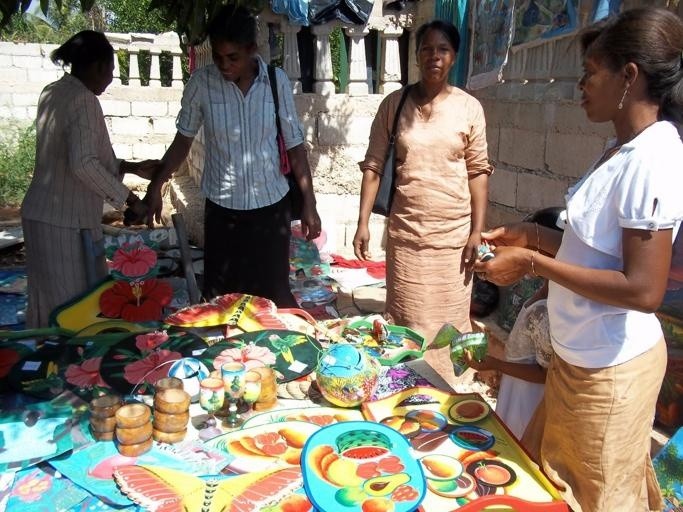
[[328, 459, 362, 487]]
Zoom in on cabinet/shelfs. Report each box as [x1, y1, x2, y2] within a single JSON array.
[[651, 288, 683, 447]]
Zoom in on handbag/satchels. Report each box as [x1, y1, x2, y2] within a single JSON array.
[[372, 84, 411, 217], [284, 168, 305, 221], [277, 134, 292, 176]]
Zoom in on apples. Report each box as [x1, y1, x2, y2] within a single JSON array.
[[474, 463, 511, 486]]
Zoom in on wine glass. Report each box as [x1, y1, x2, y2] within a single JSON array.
[[200, 361, 261, 427]]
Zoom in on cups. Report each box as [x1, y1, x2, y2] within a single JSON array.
[[250, 365, 277, 412], [89, 377, 191, 457]]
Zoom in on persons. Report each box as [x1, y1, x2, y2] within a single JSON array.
[[458, 206, 565, 441], [461, 6, 682, 512], [115, 5, 323, 318], [17, 30, 173, 376], [352, 20, 493, 389]]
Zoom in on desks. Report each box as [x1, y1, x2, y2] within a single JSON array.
[[0, 313, 456, 512]]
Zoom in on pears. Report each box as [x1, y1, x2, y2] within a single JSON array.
[[379, 411, 440, 436]]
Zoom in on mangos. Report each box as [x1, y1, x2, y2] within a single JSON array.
[[284, 448, 300, 465], [278, 429, 303, 450], [422, 459, 454, 478]]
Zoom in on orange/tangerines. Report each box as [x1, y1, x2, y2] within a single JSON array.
[[335, 486, 368, 506]]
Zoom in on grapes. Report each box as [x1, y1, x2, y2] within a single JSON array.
[[392, 485, 419, 501]]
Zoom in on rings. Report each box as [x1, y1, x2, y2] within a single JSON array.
[[481, 272, 485, 281], [317, 231, 319, 233]]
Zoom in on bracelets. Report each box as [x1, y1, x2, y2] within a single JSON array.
[[534, 221, 540, 250], [531, 251, 542, 277], [125, 194, 139, 207]]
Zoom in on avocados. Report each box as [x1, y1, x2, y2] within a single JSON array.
[[363, 474, 412, 496]]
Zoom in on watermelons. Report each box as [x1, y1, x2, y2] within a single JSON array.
[[336, 428, 391, 460]]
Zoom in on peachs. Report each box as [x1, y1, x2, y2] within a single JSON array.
[[362, 497, 396, 512], [281, 494, 314, 512]]
